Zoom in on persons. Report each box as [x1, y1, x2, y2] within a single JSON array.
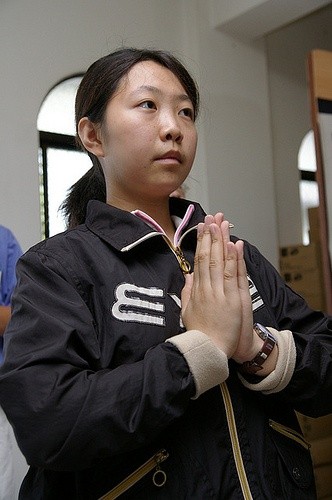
[[0, 225, 23, 351], [0, 48, 332, 500]]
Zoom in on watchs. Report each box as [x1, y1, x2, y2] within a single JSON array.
[[238, 322, 276, 375]]
